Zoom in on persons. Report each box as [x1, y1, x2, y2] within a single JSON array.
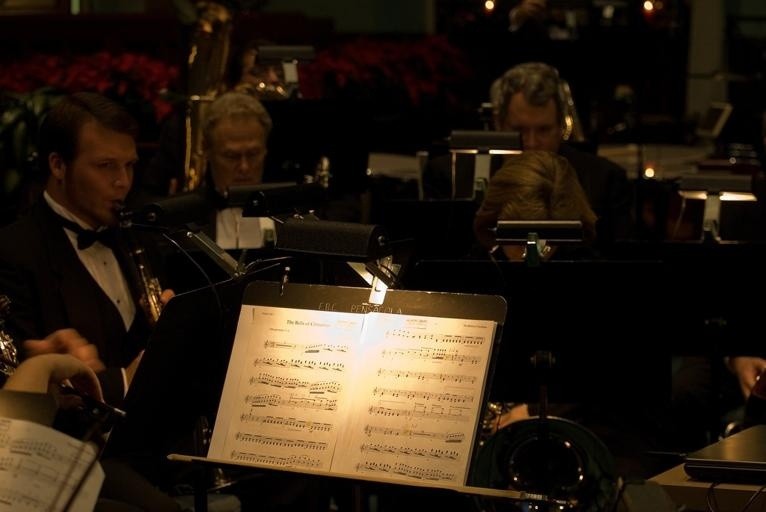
[[723, 355, 766, 402], [422, 61, 629, 242], [473, 149, 604, 260], [141, 87, 277, 240], [1, 326, 106, 406], [1, 90, 177, 409], [508, 0, 562, 62]]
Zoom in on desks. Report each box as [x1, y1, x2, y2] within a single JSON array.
[[365, 148, 749, 226], [642, 461, 765, 509]]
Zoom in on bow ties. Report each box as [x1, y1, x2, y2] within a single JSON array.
[[77, 229, 116, 250]]
